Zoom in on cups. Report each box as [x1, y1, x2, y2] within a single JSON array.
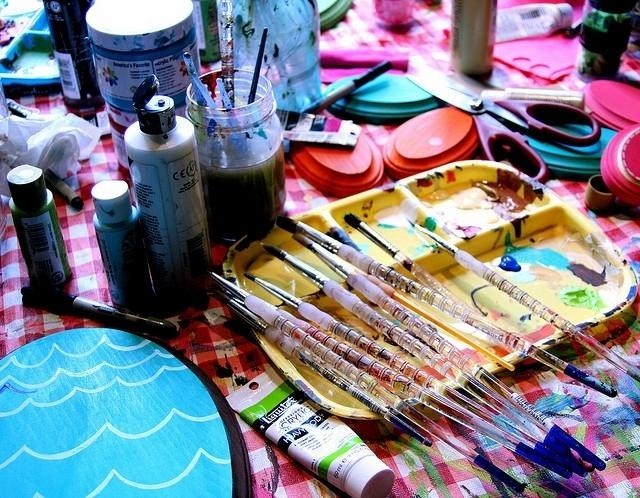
[[183, 67, 288, 246]]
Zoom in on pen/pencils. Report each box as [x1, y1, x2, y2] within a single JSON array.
[[20, 285, 177, 339], [299, 61, 393, 114], [44, 167, 85, 211]]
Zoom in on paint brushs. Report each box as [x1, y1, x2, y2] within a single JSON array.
[[182, 27, 268, 156], [206, 206, 640, 493]]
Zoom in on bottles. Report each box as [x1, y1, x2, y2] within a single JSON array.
[[123, 74, 214, 296], [91, 179, 154, 309], [4, 164, 72, 289]]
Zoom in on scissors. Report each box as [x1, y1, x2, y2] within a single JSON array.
[[405, 66, 601, 185]]
